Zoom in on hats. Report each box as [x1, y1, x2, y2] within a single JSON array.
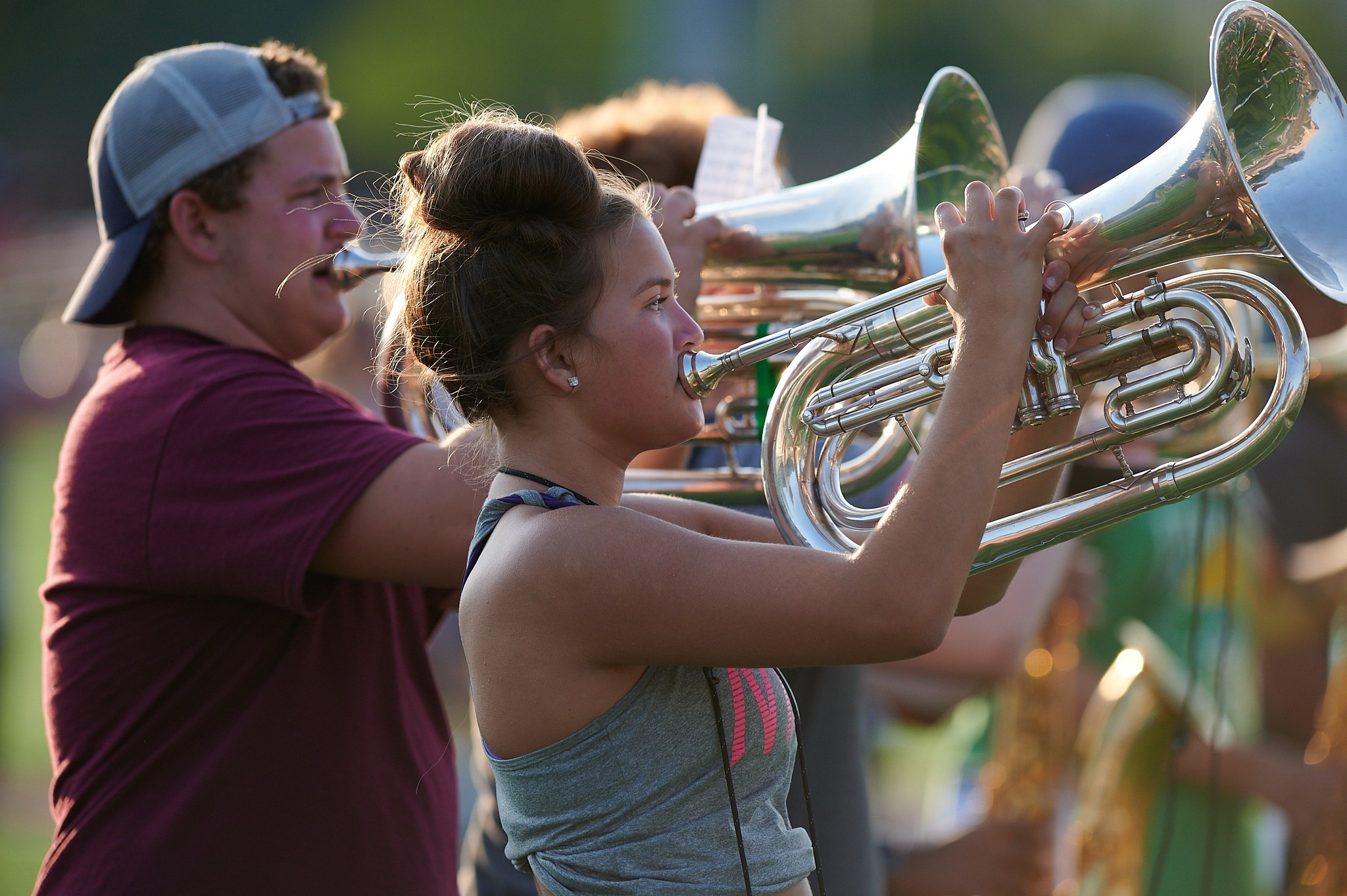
[[1013, 73, 1196, 198], [60, 43, 327, 326]]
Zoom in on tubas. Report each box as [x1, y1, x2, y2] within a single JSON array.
[[677, 0, 1347, 575], [327, 67, 1013, 506]]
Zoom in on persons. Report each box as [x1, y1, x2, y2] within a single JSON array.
[[458, 76, 1347, 896], [402, 120, 1106, 896], [34, 38, 722, 895]]
